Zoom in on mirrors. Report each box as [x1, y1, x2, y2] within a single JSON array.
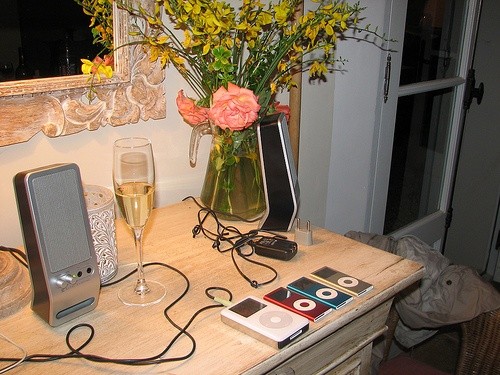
[[0, 2, 166, 149]]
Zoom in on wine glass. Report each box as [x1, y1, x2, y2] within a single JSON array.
[[113, 137, 168, 306]]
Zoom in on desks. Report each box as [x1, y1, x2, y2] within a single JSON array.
[[0, 187, 431, 374]]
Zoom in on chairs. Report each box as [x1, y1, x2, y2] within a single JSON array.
[[348, 227, 500, 375]]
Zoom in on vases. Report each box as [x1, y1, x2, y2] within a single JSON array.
[[198, 106, 270, 222]]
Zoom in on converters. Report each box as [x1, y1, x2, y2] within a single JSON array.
[[255, 236, 298, 261]]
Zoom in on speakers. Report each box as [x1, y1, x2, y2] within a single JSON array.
[[257, 112, 302, 232], [14, 162, 102, 326]]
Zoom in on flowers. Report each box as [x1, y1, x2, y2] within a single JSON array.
[[75, 0, 403, 106]]
